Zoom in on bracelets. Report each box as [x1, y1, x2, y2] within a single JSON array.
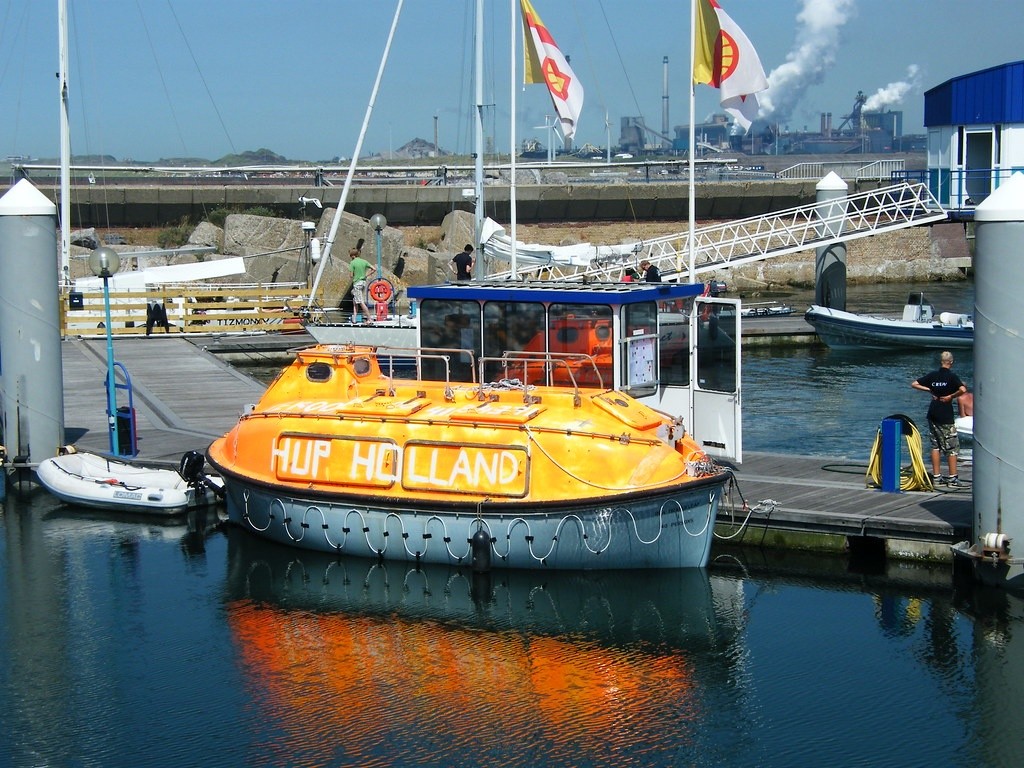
[[453, 271, 455, 274]]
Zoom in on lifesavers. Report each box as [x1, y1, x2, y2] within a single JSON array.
[[370, 281, 392, 302]]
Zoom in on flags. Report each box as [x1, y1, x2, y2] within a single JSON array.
[[692, 0, 769, 136], [520, 0, 584, 140]]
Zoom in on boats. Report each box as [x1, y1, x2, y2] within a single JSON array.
[[805, 292, 974, 352], [207, 342, 733, 569], [36, 450, 224, 515]]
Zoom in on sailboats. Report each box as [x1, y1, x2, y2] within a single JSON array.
[[299, 1, 696, 357]]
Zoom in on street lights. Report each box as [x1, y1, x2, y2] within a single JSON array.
[[87, 244, 122, 459], [367, 212, 389, 326]]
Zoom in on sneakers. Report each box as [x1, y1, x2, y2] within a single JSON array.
[[934, 474, 948, 485], [947, 475, 970, 489]]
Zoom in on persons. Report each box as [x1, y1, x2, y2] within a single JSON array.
[[910, 351, 970, 488], [623, 260, 662, 285], [349, 250, 376, 324], [437, 314, 537, 384], [957, 381, 974, 417], [447, 244, 476, 286]]
